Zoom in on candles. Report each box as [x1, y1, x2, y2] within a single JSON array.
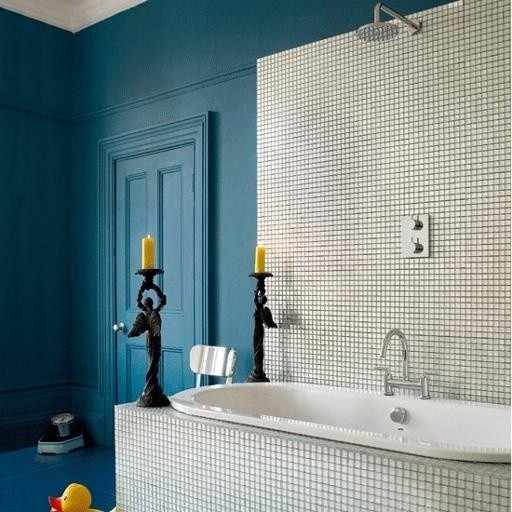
[[142, 234, 155, 269], [255, 245, 266, 273]]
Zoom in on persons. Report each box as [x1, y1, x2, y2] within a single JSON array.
[[253, 288, 268, 375], [136, 281, 167, 395]]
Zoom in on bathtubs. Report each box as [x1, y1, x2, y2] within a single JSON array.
[[168, 378, 511, 466]]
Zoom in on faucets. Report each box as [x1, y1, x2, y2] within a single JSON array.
[[379, 326, 411, 397]]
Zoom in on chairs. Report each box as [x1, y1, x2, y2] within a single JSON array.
[[189, 344, 237, 387]]
